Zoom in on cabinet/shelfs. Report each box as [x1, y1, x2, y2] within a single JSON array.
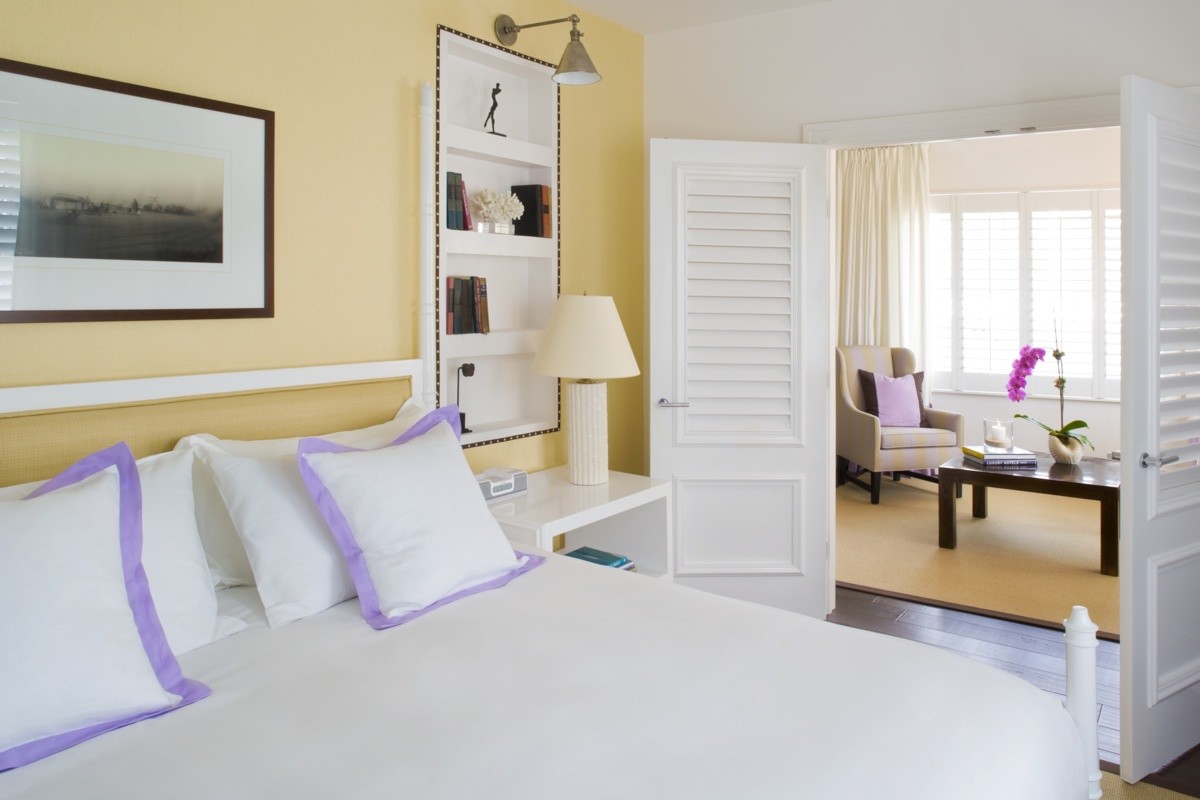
[[422, 22, 561, 448]]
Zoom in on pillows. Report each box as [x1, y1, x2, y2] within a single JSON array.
[[0, 450, 246, 657], [296, 402, 548, 630], [175, 436, 254, 586], [858, 368, 931, 428], [189, 397, 359, 626], [0, 441, 212, 775]]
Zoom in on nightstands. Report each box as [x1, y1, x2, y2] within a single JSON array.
[[487, 463, 673, 580]]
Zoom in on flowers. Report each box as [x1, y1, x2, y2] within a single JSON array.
[[1005, 346, 1095, 450], [471, 189, 525, 222]]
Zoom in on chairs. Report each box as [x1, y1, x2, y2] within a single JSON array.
[[836, 345, 965, 505]]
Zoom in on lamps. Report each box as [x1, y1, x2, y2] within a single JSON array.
[[494, 12, 603, 85], [529, 291, 641, 486], [456, 363, 475, 434]]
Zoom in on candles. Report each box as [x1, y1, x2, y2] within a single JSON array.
[[991, 419, 1007, 442]]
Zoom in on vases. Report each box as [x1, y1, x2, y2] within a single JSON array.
[[476, 221, 516, 235], [1049, 434, 1085, 464]]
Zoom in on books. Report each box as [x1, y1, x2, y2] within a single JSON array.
[[446, 172, 473, 231], [447, 276, 490, 334], [511, 184, 552, 238], [565, 546, 638, 573], [962, 446, 1038, 465]]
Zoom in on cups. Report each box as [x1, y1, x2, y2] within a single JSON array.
[[982, 418, 1013, 454]]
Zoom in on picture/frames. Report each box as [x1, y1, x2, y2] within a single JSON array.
[[1, 56, 275, 324]]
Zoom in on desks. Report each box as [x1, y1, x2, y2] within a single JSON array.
[[938, 447, 1120, 577]]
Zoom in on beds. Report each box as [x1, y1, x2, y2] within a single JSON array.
[[0, 547, 1103, 800]]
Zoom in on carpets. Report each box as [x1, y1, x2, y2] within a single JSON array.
[[1100, 768, 1200, 800], [836, 471, 1121, 633]]
